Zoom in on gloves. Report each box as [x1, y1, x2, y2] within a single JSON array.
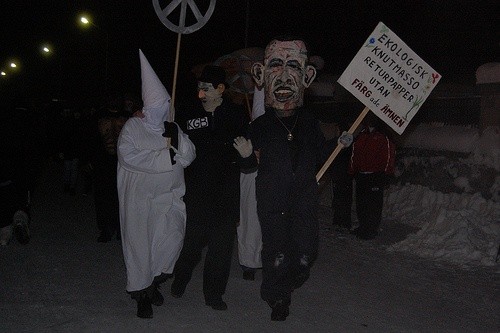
[[169, 148, 176, 165], [233, 137, 253, 159], [162, 121, 178, 147], [338, 132, 354, 147]]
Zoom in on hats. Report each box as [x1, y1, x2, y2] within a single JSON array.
[[197, 65, 230, 88]]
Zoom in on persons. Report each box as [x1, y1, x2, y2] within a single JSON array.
[[0, 94, 38, 249], [325, 124, 354, 231], [117, 56, 196, 318], [233, 35, 354, 320], [90, 95, 130, 243], [350, 120, 396, 237], [133, 65, 259, 311]]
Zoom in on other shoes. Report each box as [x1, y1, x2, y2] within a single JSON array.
[[117, 231, 121, 240], [205, 295, 227, 311], [151, 289, 164, 306], [262, 291, 289, 321], [170, 277, 188, 298], [97, 228, 115, 243], [243, 270, 254, 280], [137, 292, 153, 318], [350, 227, 378, 240]]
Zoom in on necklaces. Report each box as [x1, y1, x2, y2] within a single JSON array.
[[276, 115, 298, 141]]
